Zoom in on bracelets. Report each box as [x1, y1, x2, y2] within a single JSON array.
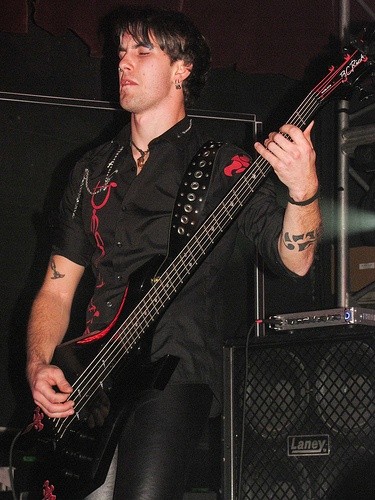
[[287, 183, 321, 206]]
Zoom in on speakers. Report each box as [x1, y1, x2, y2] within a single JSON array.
[[222, 328, 375, 500]]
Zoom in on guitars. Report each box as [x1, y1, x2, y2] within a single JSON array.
[[14, 27, 375, 500]]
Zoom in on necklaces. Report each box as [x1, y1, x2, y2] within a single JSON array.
[[128, 136, 150, 168]]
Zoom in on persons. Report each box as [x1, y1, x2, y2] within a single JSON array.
[[23, 9, 322, 500]]
